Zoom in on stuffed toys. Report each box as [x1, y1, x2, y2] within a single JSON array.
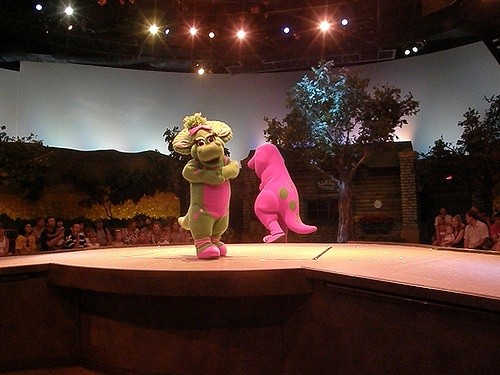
[[173, 111, 240, 259], [247, 144, 317, 243]]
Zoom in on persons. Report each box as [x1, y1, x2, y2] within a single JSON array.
[[433, 197, 500, 252], [0, 217, 233, 257]]
